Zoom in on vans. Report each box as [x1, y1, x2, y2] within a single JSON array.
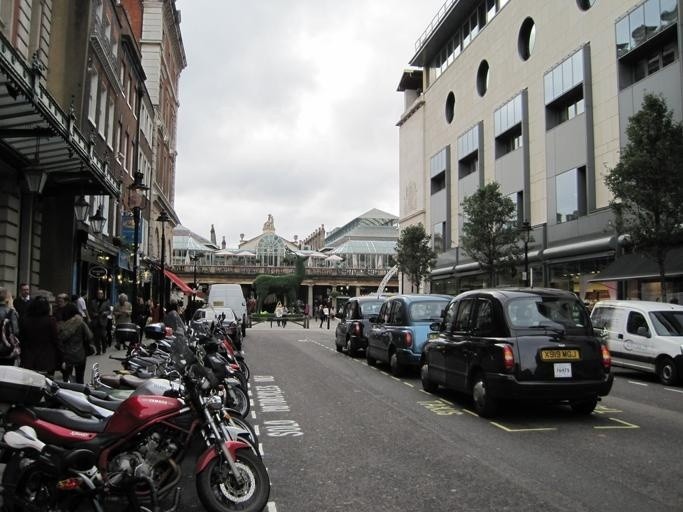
[[205, 284, 248, 337]]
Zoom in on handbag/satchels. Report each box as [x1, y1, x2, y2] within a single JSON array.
[[87, 344, 97, 356]]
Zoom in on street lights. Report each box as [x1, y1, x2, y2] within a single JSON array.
[[517, 218, 532, 286], [127, 169, 151, 326], [157, 207, 169, 323]]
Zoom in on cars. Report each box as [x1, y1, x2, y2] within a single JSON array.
[[191, 308, 242, 350]]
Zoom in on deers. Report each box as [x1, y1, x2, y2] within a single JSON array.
[[252, 247, 311, 316]]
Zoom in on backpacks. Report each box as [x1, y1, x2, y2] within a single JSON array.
[[0, 309, 20, 360]]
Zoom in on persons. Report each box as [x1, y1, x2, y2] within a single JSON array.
[[0, 282, 203, 385], [247, 291, 256, 313], [273, 299, 337, 328]]
